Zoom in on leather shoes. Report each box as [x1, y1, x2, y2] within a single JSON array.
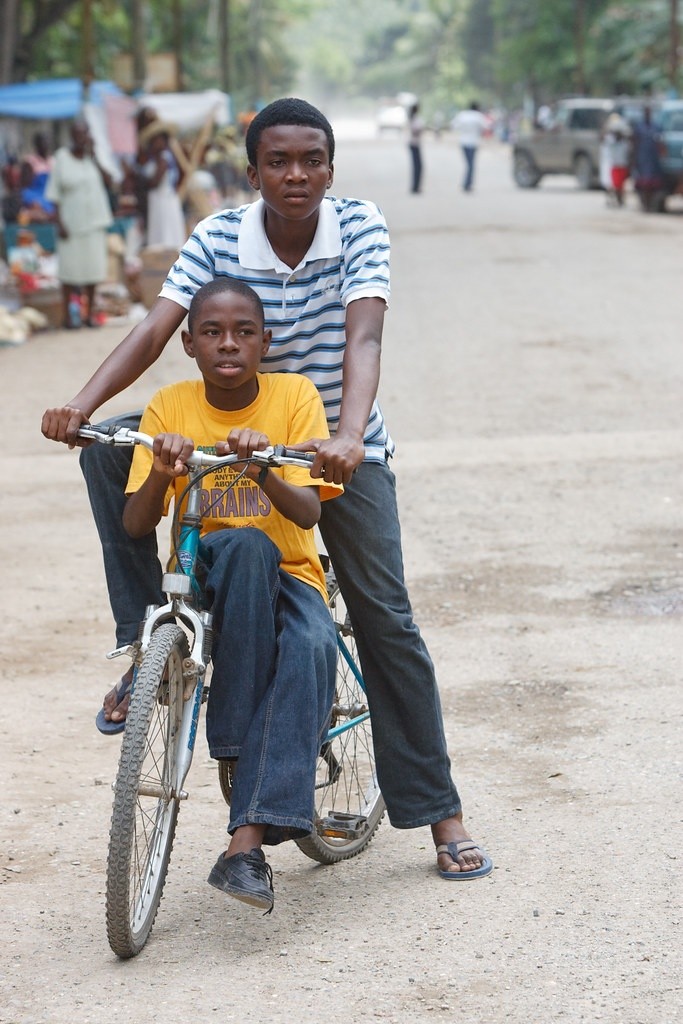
[[207, 846, 275, 918], [218, 758, 238, 806]]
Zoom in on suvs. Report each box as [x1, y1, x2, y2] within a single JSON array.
[[623, 97, 683, 194], [510, 96, 616, 189]]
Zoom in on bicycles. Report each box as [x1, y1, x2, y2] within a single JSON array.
[[67, 415, 388, 958]]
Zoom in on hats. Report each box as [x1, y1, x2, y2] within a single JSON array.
[[138, 120, 176, 148]]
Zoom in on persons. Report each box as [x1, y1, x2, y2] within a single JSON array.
[[599, 103, 665, 212], [43, 107, 186, 331], [405, 100, 428, 194], [39, 97, 494, 883], [120, 278, 347, 915], [450, 100, 493, 193]]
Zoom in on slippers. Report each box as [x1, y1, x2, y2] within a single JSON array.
[[96, 661, 168, 736], [437, 839, 492, 880]]
